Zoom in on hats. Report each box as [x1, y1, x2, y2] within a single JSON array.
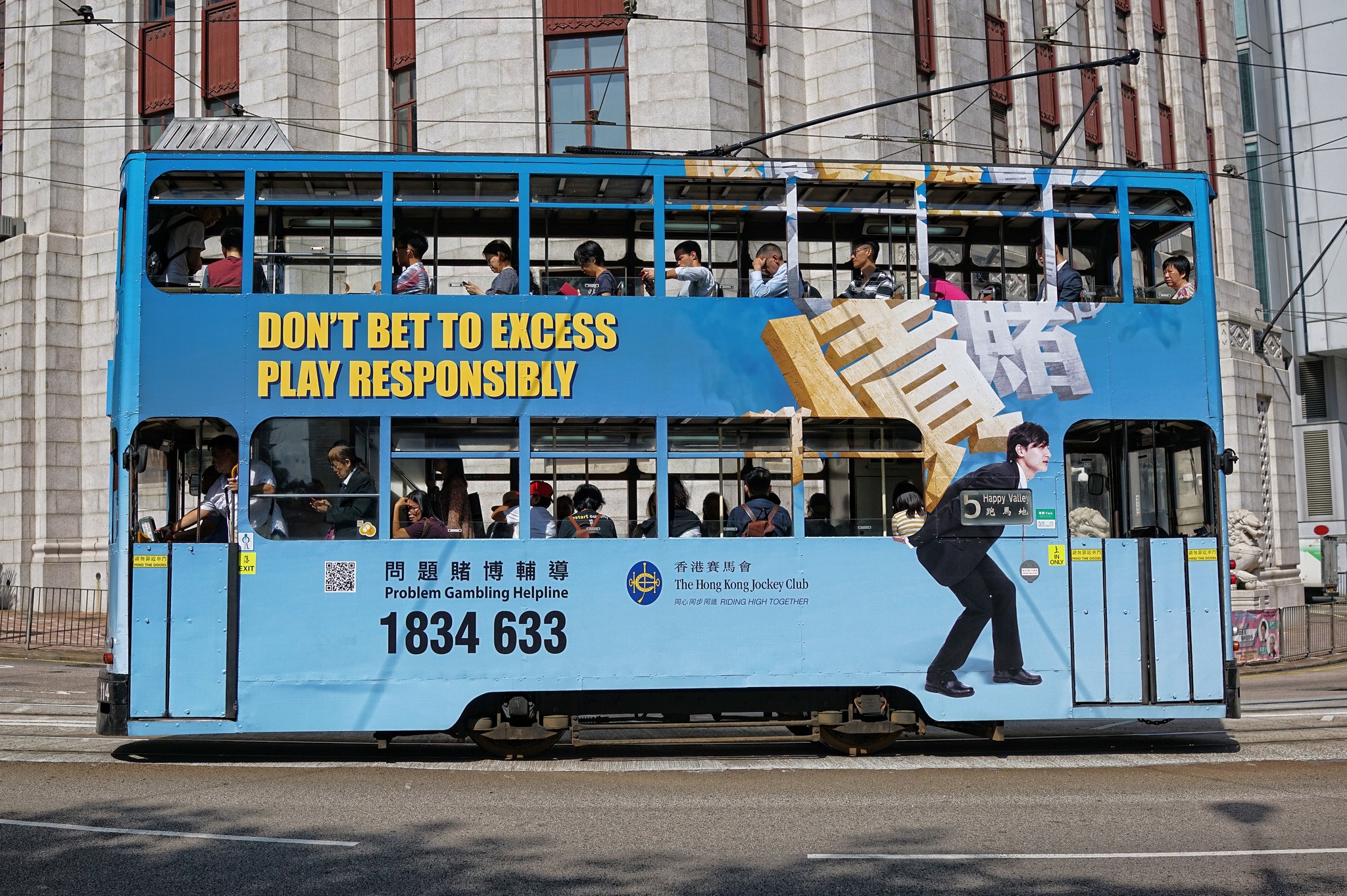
[[491, 491, 519, 512], [516, 481, 553, 498]]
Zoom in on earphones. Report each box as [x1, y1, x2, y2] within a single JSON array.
[[406, 250, 409, 253]]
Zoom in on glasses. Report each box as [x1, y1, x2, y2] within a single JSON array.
[[402, 506, 420, 515], [395, 247, 415, 253], [201, 476, 209, 482], [851, 247, 869, 255], [331, 459, 345, 470]]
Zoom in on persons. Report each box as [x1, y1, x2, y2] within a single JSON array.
[[1261, 621, 1266, 643], [574, 241, 617, 296], [392, 440, 474, 539], [374, 231, 429, 295], [1162, 255, 1196, 300], [1030, 233, 1083, 302], [633, 473, 703, 538], [892, 480, 926, 536], [929, 261, 970, 300], [804, 493, 835, 537], [156, 195, 226, 288], [157, 464, 228, 543], [901, 422, 1052, 698], [279, 438, 379, 540], [836, 237, 893, 299], [726, 466, 793, 537], [153, 435, 288, 540], [1268, 636, 1276, 657], [641, 241, 716, 297], [488, 480, 617, 538], [202, 227, 269, 294], [750, 243, 804, 298], [464, 240, 519, 295], [703, 491, 731, 537]]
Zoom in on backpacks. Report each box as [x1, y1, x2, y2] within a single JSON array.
[[568, 514, 603, 538], [738, 504, 780, 537], [146, 216, 204, 284]]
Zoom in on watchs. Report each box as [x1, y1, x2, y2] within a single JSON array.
[[297, 511, 301, 517]]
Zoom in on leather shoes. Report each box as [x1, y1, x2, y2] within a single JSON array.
[[925, 673, 975, 698], [993, 668, 1043, 685]]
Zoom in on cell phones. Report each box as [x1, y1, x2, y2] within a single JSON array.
[[310, 497, 319, 503], [462, 281, 469, 285], [555, 291, 565, 295], [639, 272, 651, 283]]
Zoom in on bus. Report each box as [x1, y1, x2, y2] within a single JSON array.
[[94, 48, 1245, 763]]
